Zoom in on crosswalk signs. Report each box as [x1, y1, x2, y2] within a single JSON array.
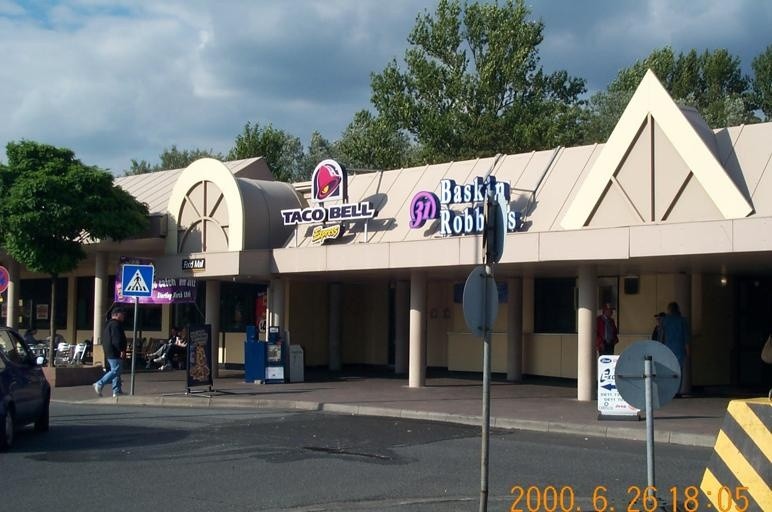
[[122, 264, 154, 297]]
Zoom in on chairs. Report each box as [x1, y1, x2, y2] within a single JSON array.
[[27, 336, 187, 372]]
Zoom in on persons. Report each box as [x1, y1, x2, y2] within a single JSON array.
[[595, 304, 619, 355], [93, 307, 127, 398], [23, 326, 41, 345], [652, 302, 690, 398], [147, 326, 188, 371]]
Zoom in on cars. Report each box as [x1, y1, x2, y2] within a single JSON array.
[[0, 326, 50, 448]]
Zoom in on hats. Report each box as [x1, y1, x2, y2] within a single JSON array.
[[654, 313, 665, 317], [604, 304, 616, 310], [111, 308, 127, 313]]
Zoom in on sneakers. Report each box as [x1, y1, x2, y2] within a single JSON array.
[[93, 383, 103, 395], [113, 393, 127, 397], [147, 353, 168, 370]]
